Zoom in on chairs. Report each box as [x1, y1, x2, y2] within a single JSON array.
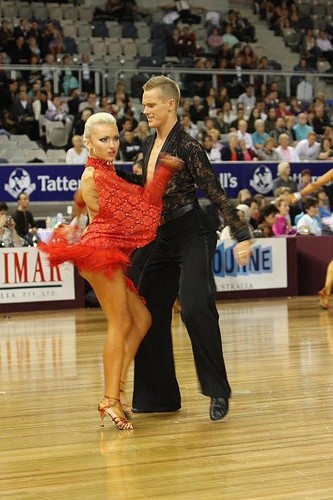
[[0, 0, 333, 165]]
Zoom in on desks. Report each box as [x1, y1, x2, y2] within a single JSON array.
[[0, 248, 86, 313], [211, 233, 333, 300]]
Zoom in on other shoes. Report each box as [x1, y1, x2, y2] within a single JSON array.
[[318, 288, 331, 309]]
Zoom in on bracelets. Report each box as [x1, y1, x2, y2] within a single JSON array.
[[294, 192, 302, 201]]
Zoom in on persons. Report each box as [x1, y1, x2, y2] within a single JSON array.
[[0, 0, 333, 313], [38, 112, 180, 430], [131, 76, 232, 421]]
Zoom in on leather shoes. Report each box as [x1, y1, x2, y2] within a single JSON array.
[[210, 397, 228, 420], [158, 201, 200, 227]]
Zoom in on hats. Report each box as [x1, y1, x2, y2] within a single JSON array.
[[312, 91, 325, 98], [131, 406, 160, 413]]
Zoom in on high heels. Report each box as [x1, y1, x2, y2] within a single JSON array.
[[98, 397, 135, 431]]
